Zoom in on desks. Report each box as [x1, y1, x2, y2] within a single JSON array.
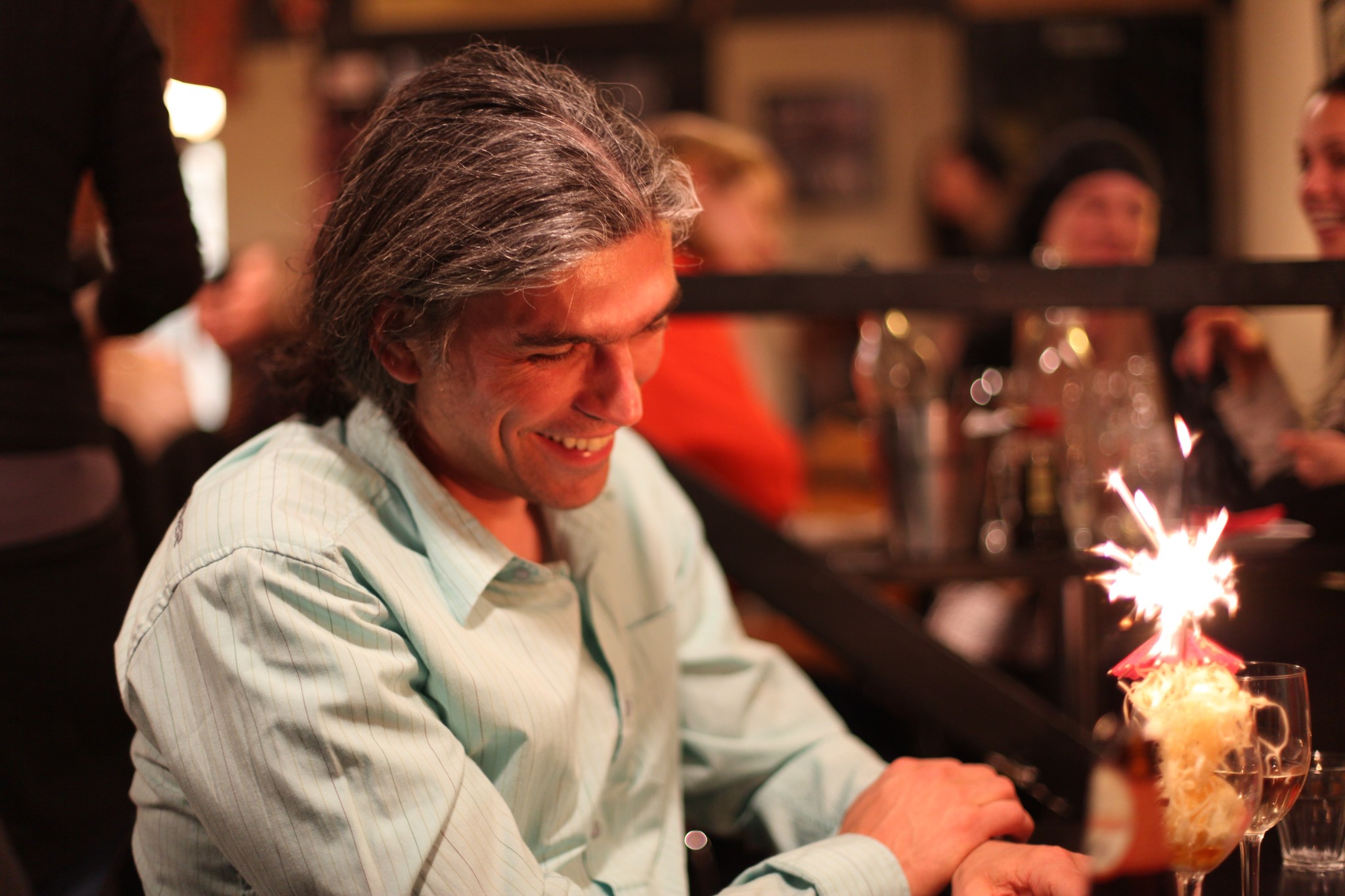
[[825, 517, 1315, 740]]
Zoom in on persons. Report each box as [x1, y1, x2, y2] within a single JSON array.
[[109, 33, 1098, 896], [942, 121, 1259, 514], [67, 151, 360, 559], [1165, 77, 1345, 577], [619, 105, 815, 534], [914, 103, 1056, 380], [0, 0, 207, 896]]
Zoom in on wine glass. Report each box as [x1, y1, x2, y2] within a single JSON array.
[[1122, 687, 1264, 896], [1230, 661, 1314, 895]]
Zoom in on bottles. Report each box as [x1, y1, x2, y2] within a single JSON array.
[[843, 251, 1194, 562]]
